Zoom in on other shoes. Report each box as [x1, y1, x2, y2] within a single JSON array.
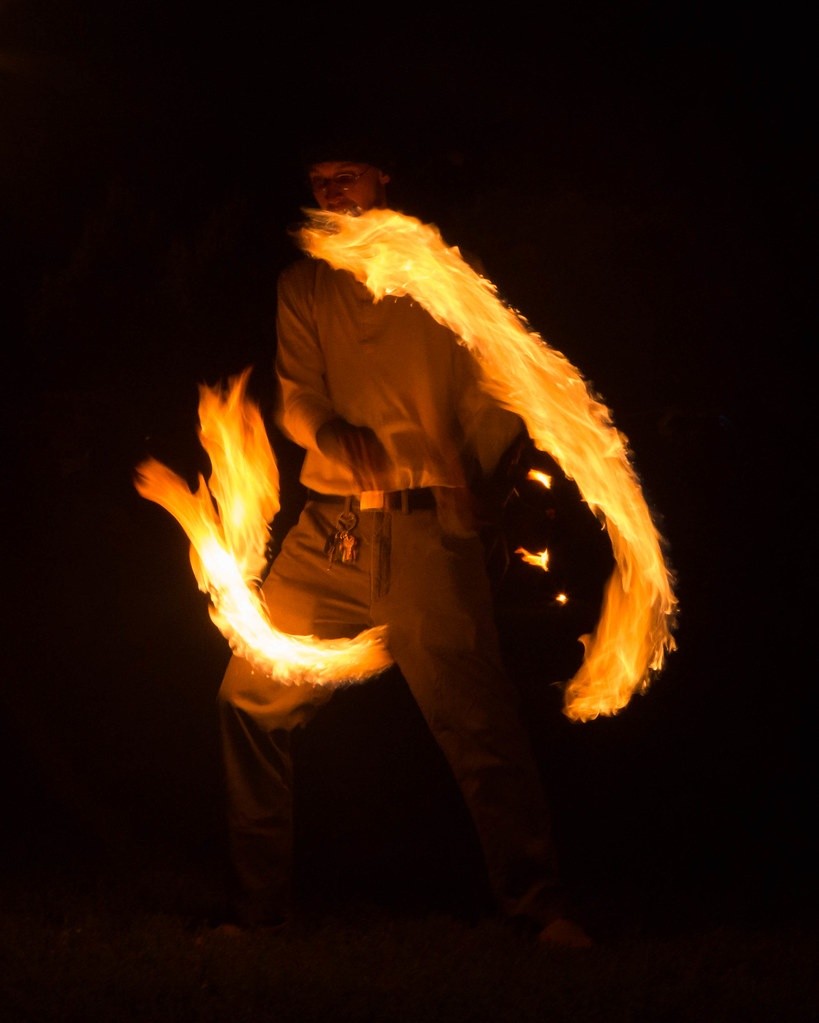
[[191, 906, 294, 946], [505, 907, 592, 952]]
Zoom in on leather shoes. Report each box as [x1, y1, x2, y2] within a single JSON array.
[[307, 487, 480, 510]]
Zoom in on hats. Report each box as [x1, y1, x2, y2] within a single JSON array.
[[305, 128, 401, 177]]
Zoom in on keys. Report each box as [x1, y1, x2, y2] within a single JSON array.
[[324, 529, 357, 569]]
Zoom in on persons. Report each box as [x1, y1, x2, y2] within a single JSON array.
[[182, 125, 588, 960]]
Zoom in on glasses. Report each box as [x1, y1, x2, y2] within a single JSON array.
[[305, 167, 371, 189]]
